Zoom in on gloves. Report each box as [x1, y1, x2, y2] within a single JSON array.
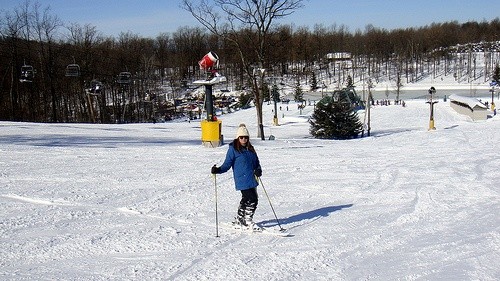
[[255, 169, 262, 177], [211, 166, 221, 174]]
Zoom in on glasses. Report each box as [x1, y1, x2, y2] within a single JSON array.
[[239, 136, 248, 139]]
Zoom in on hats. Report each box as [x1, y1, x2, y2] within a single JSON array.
[[235, 123, 249, 139]]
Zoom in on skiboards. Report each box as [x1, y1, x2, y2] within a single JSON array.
[[227, 221, 290, 237]]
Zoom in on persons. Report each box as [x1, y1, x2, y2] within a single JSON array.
[[211, 124, 262, 230]]
